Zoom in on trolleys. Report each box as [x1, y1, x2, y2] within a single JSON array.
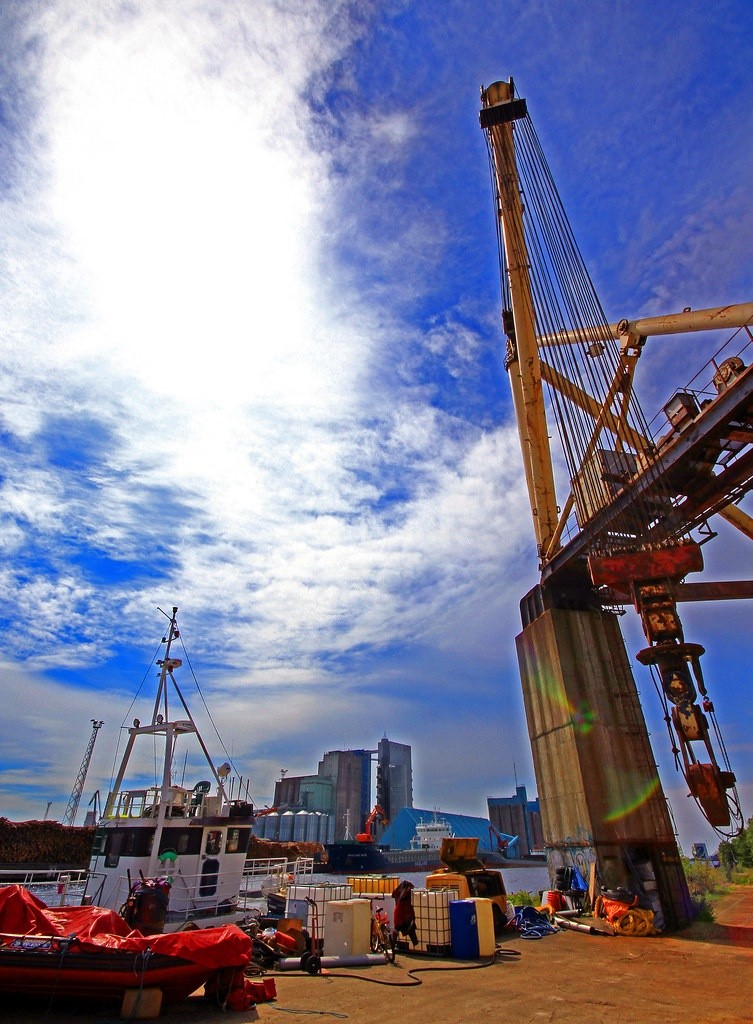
[[300, 895, 330, 975]]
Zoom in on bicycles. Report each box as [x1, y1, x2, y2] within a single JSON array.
[[364, 895, 396, 964]]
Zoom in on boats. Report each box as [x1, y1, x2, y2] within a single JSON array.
[[409, 806, 456, 849], [0, 885, 252, 1024], [82, 606, 315, 934]]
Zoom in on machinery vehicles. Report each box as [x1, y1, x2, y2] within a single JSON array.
[[356, 805, 390, 852]]
